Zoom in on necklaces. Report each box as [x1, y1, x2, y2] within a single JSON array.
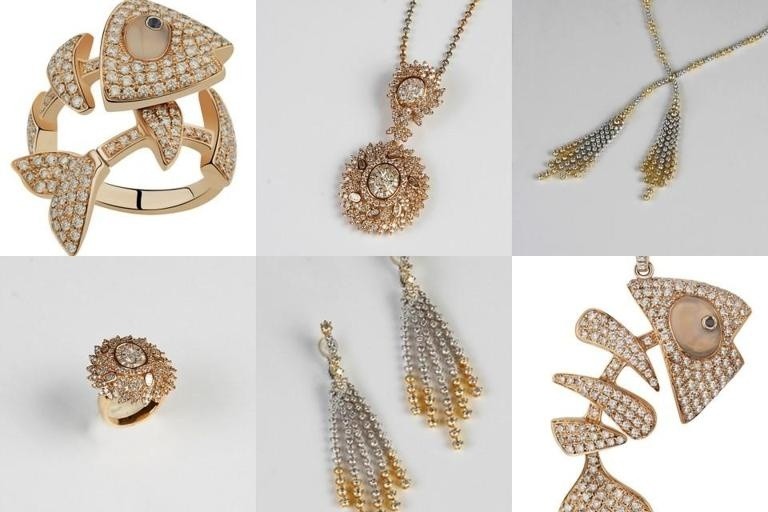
[[338, 2, 479, 235], [533, 2, 767, 201]]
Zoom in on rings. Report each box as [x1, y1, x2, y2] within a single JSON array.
[[13, 3, 236, 256], [84, 333, 178, 427]]
